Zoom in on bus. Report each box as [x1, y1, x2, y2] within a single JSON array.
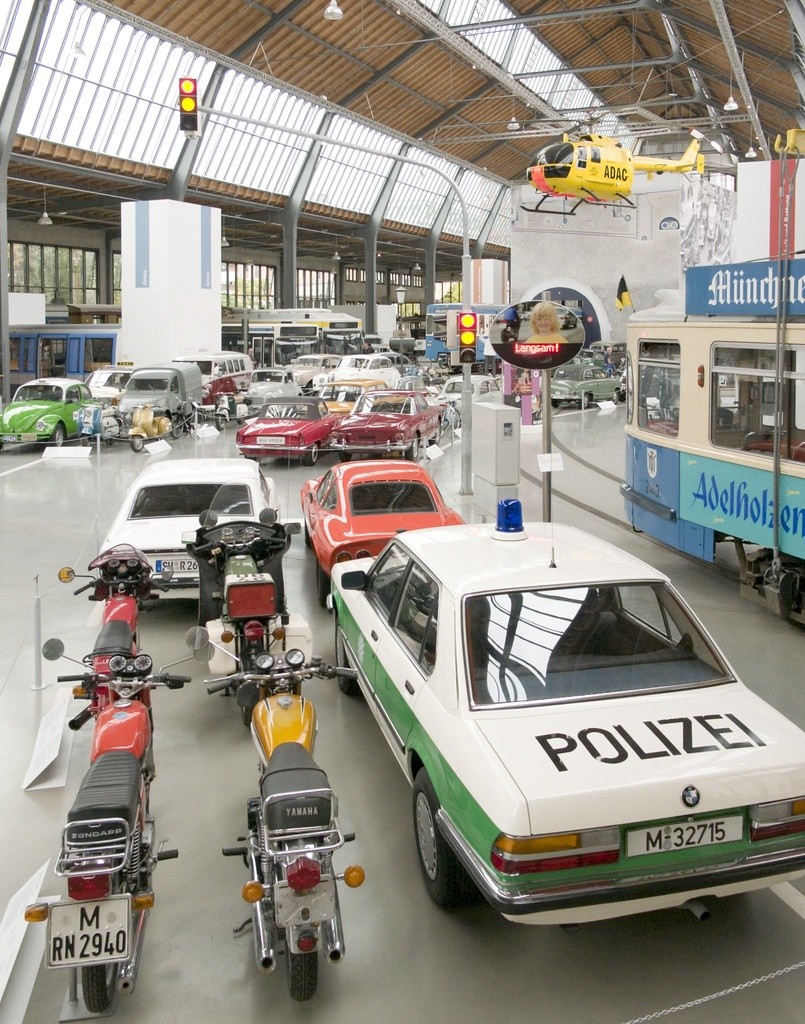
[[0, 323, 321, 401], [619, 287, 805, 626], [67, 303, 366, 356], [426, 303, 510, 375]]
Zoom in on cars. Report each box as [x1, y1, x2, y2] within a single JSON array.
[[301, 458, 466, 609], [327, 496, 805, 923], [82, 351, 503, 456], [0, 377, 102, 451], [553, 306, 584, 331], [330, 387, 448, 461], [98, 457, 278, 590], [236, 395, 346, 466], [550, 363, 624, 410], [520, 311, 531, 321]]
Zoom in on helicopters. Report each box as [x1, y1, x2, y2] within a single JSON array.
[[439, 94, 706, 216]]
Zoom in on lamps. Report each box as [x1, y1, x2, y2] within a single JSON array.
[[413, 247, 421, 270], [37, 185, 53, 226], [744, 122, 757, 159], [221, 215, 228, 246], [724, 66, 739, 112], [324, 0, 344, 21], [331, 234, 341, 260], [509, 72, 521, 130]]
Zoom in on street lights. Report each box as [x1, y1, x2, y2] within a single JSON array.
[[395, 285, 407, 377]]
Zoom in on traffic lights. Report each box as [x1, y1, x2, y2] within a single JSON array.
[[459, 313, 477, 363], [179, 77, 198, 131]]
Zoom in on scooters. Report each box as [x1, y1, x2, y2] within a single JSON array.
[[500, 320, 521, 343]]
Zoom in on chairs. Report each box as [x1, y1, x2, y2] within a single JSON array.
[[743, 431, 805, 463], [143, 495, 188, 515]]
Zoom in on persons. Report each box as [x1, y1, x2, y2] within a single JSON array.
[[605, 349, 615, 373], [363, 343, 374, 354], [503, 303, 569, 344], [512, 367, 531, 393]]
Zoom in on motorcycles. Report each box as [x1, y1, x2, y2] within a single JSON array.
[[180, 475, 304, 723], [22, 637, 215, 1013], [183, 625, 366, 1002], [58, 543, 174, 721]]
